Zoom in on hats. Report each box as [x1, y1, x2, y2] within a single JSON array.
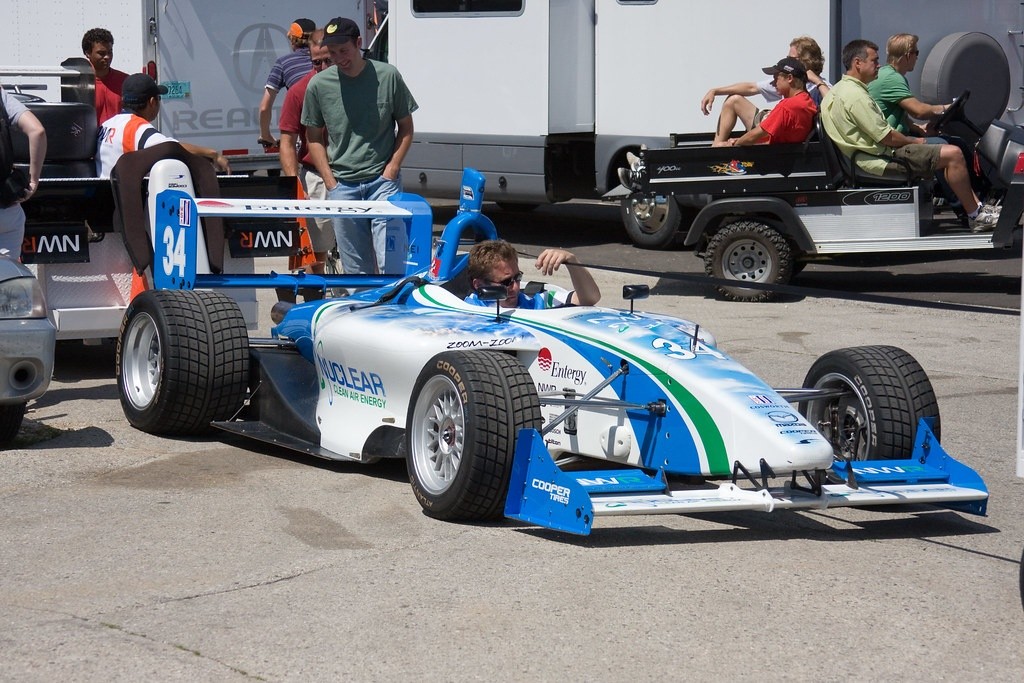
[[287, 18, 316, 39], [762, 57, 808, 82], [320, 17, 360, 49], [122, 73, 168, 99]]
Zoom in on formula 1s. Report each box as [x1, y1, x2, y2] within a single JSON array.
[[108, 167, 990, 537]]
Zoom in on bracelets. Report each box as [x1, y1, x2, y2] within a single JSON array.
[[731, 140, 737, 146], [817, 81, 824, 90]]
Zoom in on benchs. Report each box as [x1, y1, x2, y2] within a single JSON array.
[[815, 112, 922, 186]]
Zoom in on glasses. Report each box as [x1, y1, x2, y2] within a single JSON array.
[[470, 270, 523, 286], [911, 51, 919, 56], [310, 57, 332, 65]]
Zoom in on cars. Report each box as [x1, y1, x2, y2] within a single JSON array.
[[0, 253, 56, 449]]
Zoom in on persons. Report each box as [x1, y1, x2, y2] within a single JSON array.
[[82, 28, 131, 126], [258, 18, 317, 149], [819, 40, 1002, 231], [700, 36, 833, 145], [96, 73, 231, 178], [868, 33, 985, 221], [0, 85, 47, 261], [300, 16, 419, 295], [728, 57, 817, 145], [279, 28, 335, 274], [463, 238, 602, 309]]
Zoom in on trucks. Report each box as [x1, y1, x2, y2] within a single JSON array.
[[142, 0, 1024, 252]]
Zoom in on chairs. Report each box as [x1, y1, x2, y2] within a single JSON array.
[[5, 102, 99, 178]]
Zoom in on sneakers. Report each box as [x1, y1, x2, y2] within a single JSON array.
[[626, 151, 645, 172], [969, 204, 1003, 221], [617, 168, 645, 192], [964, 195, 1004, 223], [968, 212, 1000, 233]]
